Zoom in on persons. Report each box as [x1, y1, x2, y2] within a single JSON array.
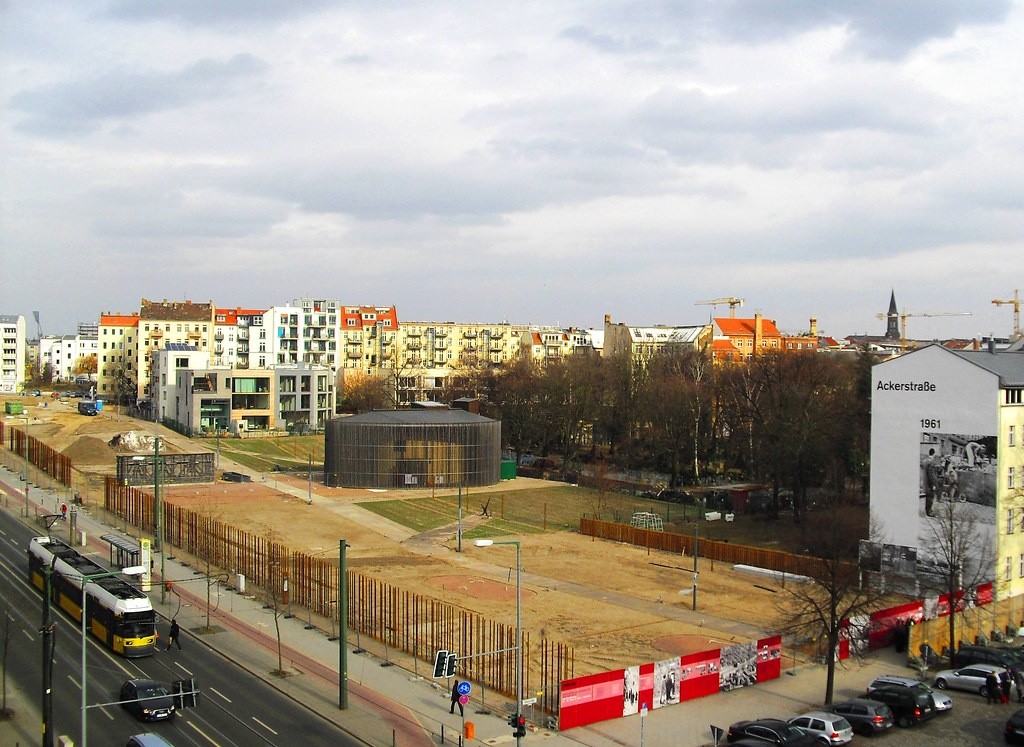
[[1000, 667, 1013, 701], [896, 615, 933, 652], [164, 620, 183, 652], [986, 671, 999, 704], [449, 680, 463, 717], [659, 670, 676, 704], [721, 649, 756, 686], [60, 503, 67, 519]]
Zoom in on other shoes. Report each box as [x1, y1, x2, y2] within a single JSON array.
[[164, 648, 169, 652], [449, 712, 454, 714], [461, 715, 463, 717], [177, 649, 183, 651]]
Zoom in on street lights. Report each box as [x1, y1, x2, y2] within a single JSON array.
[[473, 540, 523, 747], [6, 415, 29, 518], [133, 456, 164, 604], [81, 566, 148, 747]]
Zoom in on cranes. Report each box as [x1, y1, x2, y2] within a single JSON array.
[[694, 297, 746, 318], [877, 306, 973, 352], [991, 288, 1024, 334]]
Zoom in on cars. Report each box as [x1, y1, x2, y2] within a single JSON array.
[[1005, 709, 1024, 745], [816, 697, 896, 738], [120, 678, 176, 723], [934, 663, 1012, 698], [126, 732, 175, 747], [19, 389, 92, 399], [727, 718, 829, 747], [786, 710, 855, 747]]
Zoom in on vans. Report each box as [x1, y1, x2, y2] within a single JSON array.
[[77, 400, 98, 416]]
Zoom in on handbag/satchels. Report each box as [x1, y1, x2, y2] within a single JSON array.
[[168, 636, 172, 645]]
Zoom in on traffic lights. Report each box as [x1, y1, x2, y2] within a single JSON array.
[[445, 653, 458, 678], [432, 650, 448, 679], [518, 716, 526, 736]]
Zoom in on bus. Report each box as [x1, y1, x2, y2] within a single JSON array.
[[27, 536, 160, 658]]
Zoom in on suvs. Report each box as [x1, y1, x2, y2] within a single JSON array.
[[857, 684, 937, 729], [955, 645, 1024, 679], [866, 675, 953, 714]]
